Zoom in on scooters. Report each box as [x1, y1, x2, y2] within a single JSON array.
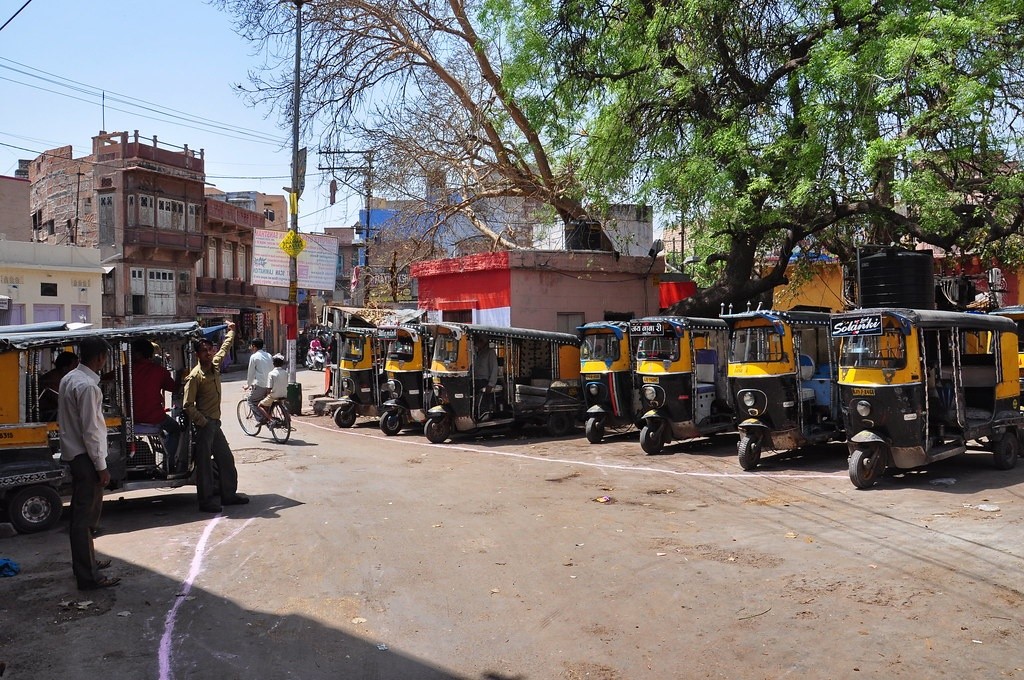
[[306, 348, 326, 371]]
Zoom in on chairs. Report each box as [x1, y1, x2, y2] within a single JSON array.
[[488, 357, 505, 405], [796, 354, 815, 416], [692, 349, 717, 424]]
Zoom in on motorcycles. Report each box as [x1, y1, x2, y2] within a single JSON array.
[[1, 320, 229, 533], [334, 322, 587, 443], [576, 301, 1024, 489]]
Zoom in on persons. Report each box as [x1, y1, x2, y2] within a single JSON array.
[[58, 336, 121, 589], [296, 332, 337, 364], [257, 352, 293, 431], [243, 338, 274, 426], [468, 335, 497, 411], [183, 320, 248, 513], [37, 352, 79, 412], [100, 338, 187, 473]]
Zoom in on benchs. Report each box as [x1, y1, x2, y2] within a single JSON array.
[[515, 368, 554, 406], [941, 365, 996, 412], [801, 363, 835, 405]]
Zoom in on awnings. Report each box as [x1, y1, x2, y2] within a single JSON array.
[[234, 307, 270, 313]]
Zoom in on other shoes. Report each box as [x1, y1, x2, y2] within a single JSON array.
[[255, 419, 267, 427], [199, 506, 222, 512], [269, 419, 277, 431], [96, 560, 111, 569], [222, 494, 249, 505], [93, 577, 121, 590]]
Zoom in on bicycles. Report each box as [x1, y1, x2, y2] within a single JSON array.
[[237, 386, 290, 443]]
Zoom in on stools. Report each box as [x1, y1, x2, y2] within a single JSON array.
[[134, 425, 169, 474]]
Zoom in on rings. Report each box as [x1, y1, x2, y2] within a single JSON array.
[[104, 480, 107, 484]]
